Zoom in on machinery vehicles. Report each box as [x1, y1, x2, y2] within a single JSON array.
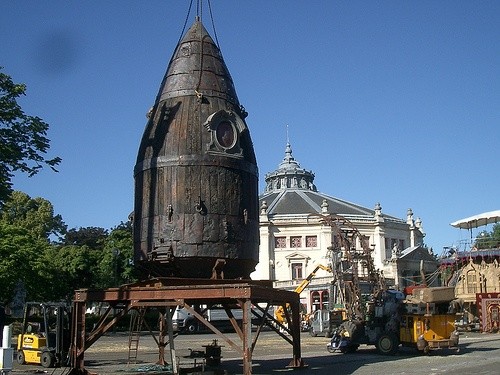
[[393, 284, 461, 355], [303, 211, 404, 355], [14, 300, 72, 368], [274, 263, 333, 333]]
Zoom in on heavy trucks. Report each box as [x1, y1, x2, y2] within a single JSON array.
[[166, 300, 269, 333]]
